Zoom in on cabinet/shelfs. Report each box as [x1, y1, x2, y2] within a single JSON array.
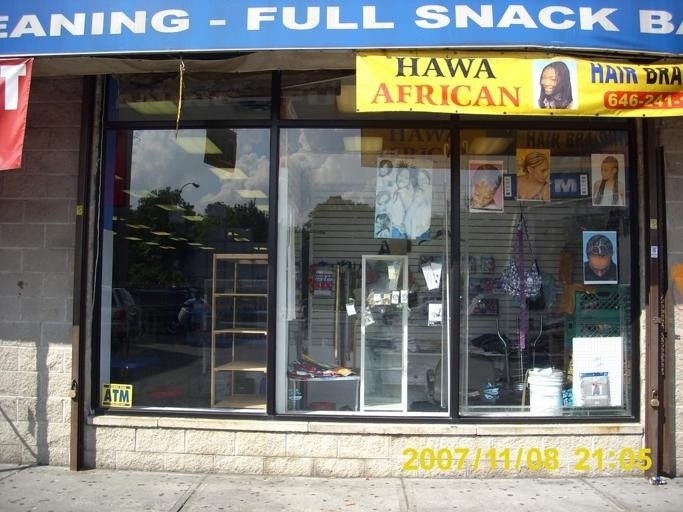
[[211, 253, 269, 410]]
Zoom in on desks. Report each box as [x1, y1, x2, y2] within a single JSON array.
[[288, 376, 360, 412]]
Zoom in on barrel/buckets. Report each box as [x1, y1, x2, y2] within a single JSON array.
[[579, 371, 610, 408], [525, 366, 566, 417]]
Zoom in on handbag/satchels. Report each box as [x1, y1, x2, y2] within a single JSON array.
[[500, 224, 544, 298]]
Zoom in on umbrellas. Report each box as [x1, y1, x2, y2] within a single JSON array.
[[340, 261, 351, 361]]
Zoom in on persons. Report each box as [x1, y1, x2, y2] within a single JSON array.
[[178, 286, 212, 346], [375, 160, 431, 239], [584, 235, 617, 281], [471, 164, 502, 209], [517, 152, 550, 200], [538, 61, 573, 109], [593, 156, 625, 205]]
